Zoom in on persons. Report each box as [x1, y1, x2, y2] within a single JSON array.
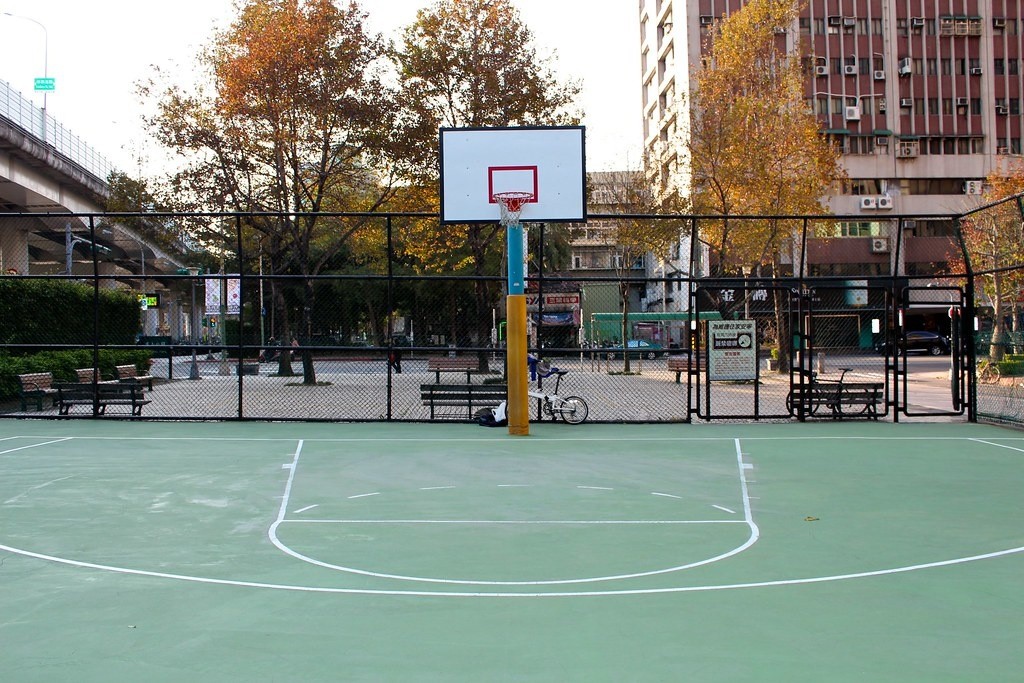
[[392, 338, 402, 373], [290, 337, 299, 354], [265, 337, 276, 358]]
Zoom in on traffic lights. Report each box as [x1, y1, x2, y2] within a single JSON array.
[[210, 318, 215, 327]]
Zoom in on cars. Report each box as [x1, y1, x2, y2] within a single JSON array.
[[600, 340, 664, 360], [874, 331, 950, 357], [384, 334, 435, 344]]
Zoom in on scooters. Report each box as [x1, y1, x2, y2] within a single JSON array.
[[258, 350, 280, 363]]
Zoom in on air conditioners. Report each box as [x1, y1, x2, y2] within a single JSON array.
[[815, 65, 885, 120], [971, 68, 982, 75], [967, 180, 982, 195], [830, 17, 855, 26], [861, 197, 916, 253], [701, 17, 712, 24], [912, 18, 924, 27], [877, 97, 920, 159], [957, 96, 1008, 156], [994, 18, 1006, 27]]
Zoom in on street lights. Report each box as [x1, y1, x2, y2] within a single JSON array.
[[927, 283, 953, 368], [654, 260, 666, 313], [185, 267, 202, 379]]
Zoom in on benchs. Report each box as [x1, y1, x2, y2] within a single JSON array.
[[793, 382, 883, 421], [668, 356, 705, 384], [426, 357, 479, 384], [17, 365, 155, 416], [420, 385, 508, 420]]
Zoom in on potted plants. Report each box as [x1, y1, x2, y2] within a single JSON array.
[[543, 356, 552, 369], [235, 360, 259, 375], [765, 349, 790, 373]]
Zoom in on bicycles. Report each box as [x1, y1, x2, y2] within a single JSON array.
[[786, 367, 870, 417], [505, 354, 588, 425], [974, 351, 1000, 384]]
